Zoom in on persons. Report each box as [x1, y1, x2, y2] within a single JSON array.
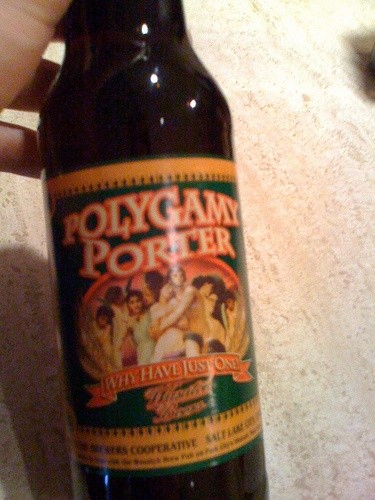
[[0, 0, 72, 180]]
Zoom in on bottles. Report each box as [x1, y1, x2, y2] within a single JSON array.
[[38, 0, 270, 500]]
